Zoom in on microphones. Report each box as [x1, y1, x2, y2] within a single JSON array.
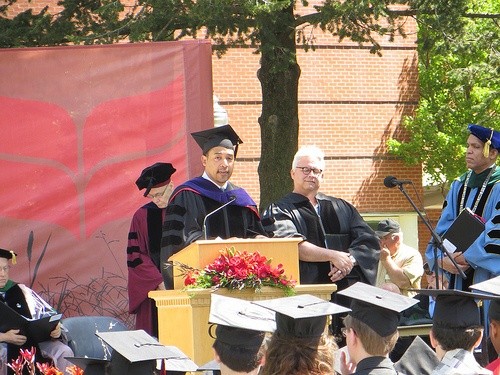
[[201, 196, 237, 240], [384, 176, 412, 188]]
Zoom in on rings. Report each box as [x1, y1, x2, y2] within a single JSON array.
[[338, 270, 342, 274]]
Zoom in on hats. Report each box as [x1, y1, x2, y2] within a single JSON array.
[[0, 249, 18, 264], [191, 124, 243, 161], [198, 359, 221, 375], [208, 293, 276, 348], [337, 282, 421, 338], [375, 219, 401, 237], [408, 289, 500, 329], [95, 329, 176, 375], [251, 294, 352, 336], [157, 345, 199, 375], [469, 275, 499, 295], [468, 123, 500, 159], [135, 162, 176, 197]]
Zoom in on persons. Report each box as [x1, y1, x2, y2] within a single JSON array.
[[63, 329, 202, 375], [160, 125, 266, 291], [261, 145, 380, 350], [128, 163, 176, 341], [426, 125, 500, 338], [0, 248, 77, 375], [374, 219, 423, 299], [401, 262, 449, 319], [196, 274, 500, 375]]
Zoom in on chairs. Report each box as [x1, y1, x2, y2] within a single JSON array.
[[61, 315, 128, 359]]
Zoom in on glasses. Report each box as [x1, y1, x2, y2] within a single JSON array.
[[296, 167, 323, 175], [147, 184, 168, 199]]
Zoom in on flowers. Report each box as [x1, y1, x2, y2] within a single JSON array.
[[163, 245, 296, 299]]
